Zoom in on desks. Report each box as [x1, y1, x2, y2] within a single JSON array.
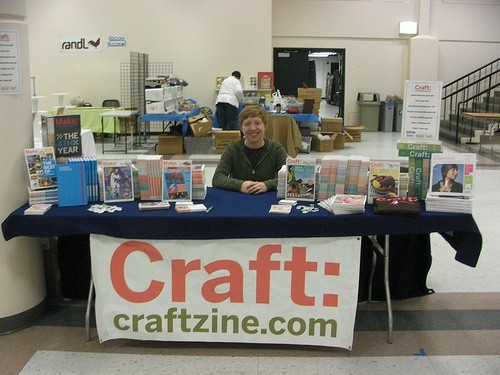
[[48, 106, 139, 155], [1, 188, 483, 343], [138, 107, 201, 149], [293, 114, 321, 128], [463, 111, 500, 155]]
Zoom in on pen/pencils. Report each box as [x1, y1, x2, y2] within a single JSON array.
[[204, 203, 215, 214]]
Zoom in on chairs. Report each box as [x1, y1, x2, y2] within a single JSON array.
[[102, 100, 120, 108], [265, 113, 303, 158]]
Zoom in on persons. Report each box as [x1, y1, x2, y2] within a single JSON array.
[[432, 164, 463, 194], [215, 71, 244, 131], [212, 105, 288, 195]]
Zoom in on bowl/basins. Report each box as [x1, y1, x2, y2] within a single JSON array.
[[371, 176, 395, 194]]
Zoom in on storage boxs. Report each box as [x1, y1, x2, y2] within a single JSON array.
[[143, 84, 242, 156], [299, 88, 366, 156]]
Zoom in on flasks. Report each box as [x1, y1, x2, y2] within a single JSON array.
[[276, 103, 281, 113]]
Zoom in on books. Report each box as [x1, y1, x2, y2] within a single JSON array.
[[24, 114, 99, 215], [99, 155, 208, 213], [269, 155, 370, 214], [367, 139, 476, 215]]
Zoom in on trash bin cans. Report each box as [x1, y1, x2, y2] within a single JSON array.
[[380, 101, 395, 132], [356, 92, 381, 131], [298, 137, 312, 154], [394, 101, 403, 132]]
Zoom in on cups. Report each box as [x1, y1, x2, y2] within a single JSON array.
[[182, 100, 195, 110]]
